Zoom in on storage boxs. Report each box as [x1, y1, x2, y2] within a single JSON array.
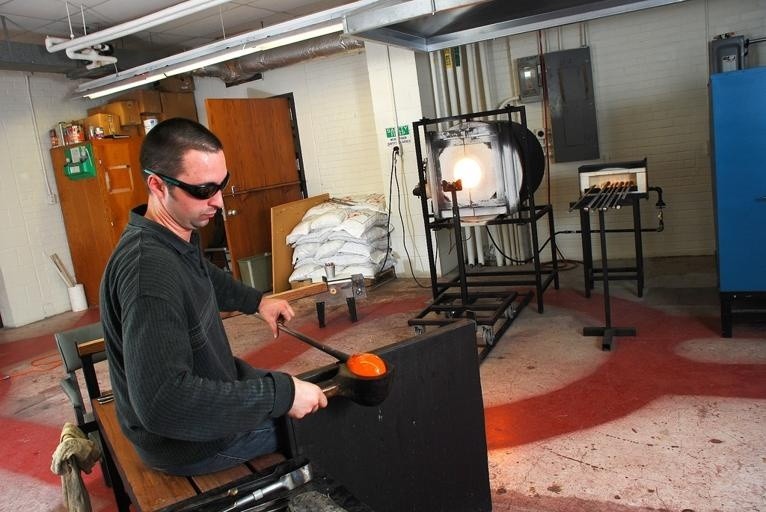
[[108, 72, 196, 116]]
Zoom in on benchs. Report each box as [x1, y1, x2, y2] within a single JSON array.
[[90, 386, 285, 511]]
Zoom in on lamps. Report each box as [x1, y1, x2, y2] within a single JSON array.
[[74, 1, 380, 101]]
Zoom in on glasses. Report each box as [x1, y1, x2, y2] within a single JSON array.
[[141, 170, 230, 199]]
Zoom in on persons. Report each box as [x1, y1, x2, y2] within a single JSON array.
[[99, 118, 328, 477]]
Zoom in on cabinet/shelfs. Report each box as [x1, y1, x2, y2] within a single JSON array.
[[48, 94, 228, 307]]
